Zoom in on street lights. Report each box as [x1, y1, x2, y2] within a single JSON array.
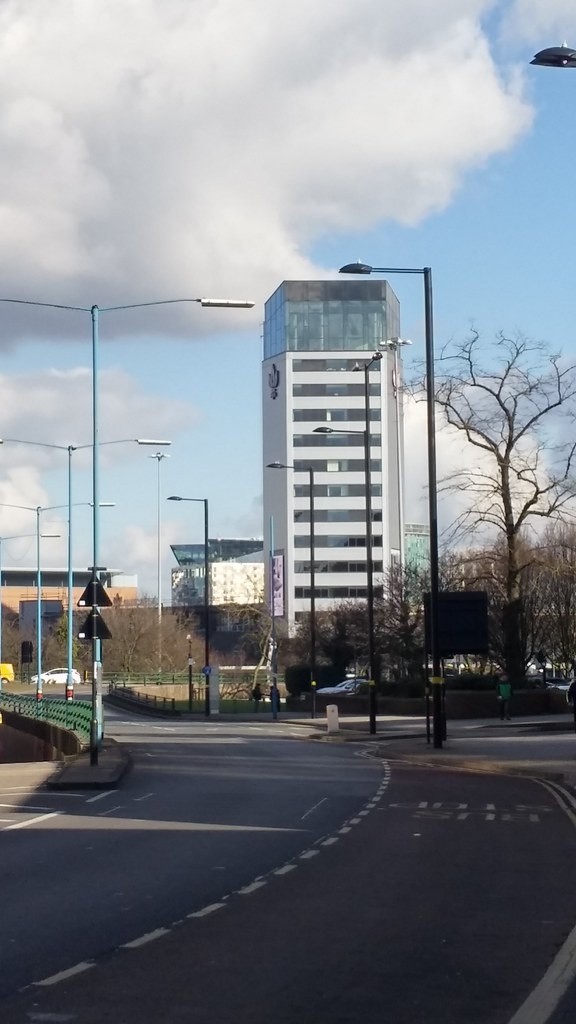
[[266, 461, 317, 719], [380, 340, 414, 620], [0, 297, 254, 775], [0, 438, 171, 727], [0, 503, 117, 721], [314, 351, 378, 738], [166, 495, 211, 717], [149, 452, 171, 683], [337, 261, 450, 750]]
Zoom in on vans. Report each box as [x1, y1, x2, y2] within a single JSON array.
[[1, 663, 14, 683]]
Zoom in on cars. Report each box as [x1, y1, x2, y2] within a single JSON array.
[[546, 682, 570, 691], [316, 678, 367, 695], [30, 667, 82, 684]]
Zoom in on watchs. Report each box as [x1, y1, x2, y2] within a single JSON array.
[[495, 675, 513, 720]]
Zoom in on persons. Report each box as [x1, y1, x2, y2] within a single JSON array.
[[252, 683, 265, 713], [566, 679, 576, 733], [265, 686, 280, 712]]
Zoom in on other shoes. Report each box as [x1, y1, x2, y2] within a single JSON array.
[[506, 717, 511, 720], [500, 716, 504, 720]]
